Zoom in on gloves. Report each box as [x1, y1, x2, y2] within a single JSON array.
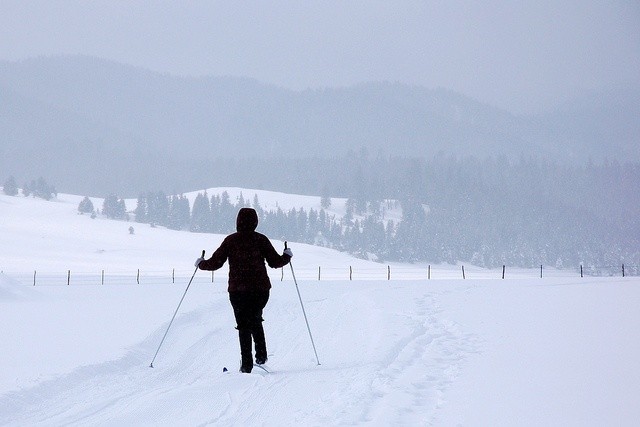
[[283, 248, 293, 257], [194, 257, 203, 268]]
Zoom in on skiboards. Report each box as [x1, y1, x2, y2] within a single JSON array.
[[222, 363, 274, 374]]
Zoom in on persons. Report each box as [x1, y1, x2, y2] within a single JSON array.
[[194, 207, 293, 372]]
